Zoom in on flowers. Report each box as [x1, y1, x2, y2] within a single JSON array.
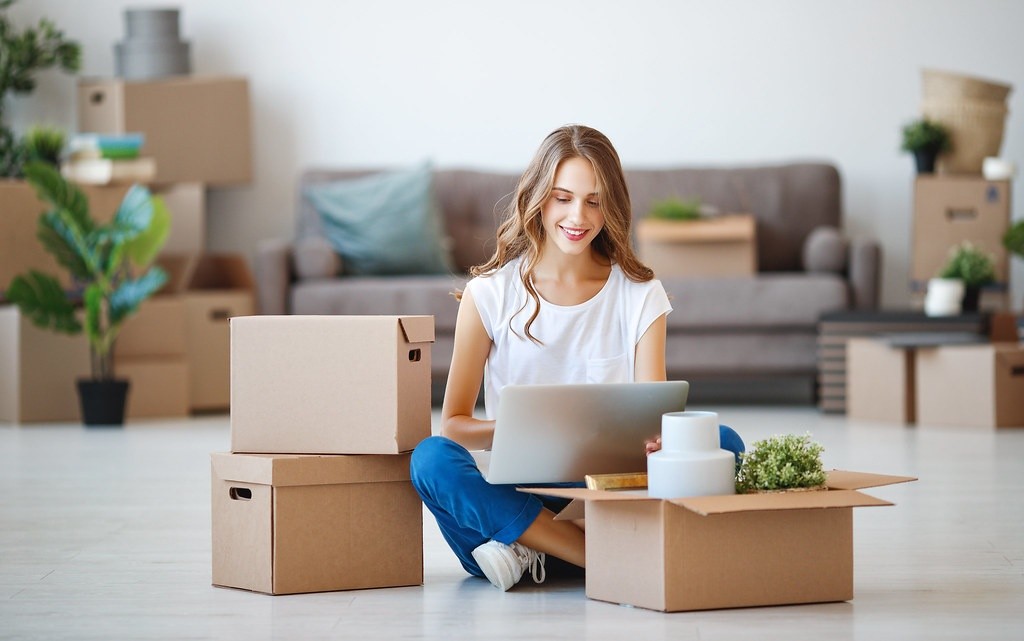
[[736, 433, 827, 485]]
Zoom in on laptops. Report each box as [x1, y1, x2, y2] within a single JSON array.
[[469, 380, 690, 484]]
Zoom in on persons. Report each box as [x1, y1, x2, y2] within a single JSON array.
[[411, 126, 744, 591]]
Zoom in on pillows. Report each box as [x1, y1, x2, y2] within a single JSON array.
[[301, 163, 453, 278]]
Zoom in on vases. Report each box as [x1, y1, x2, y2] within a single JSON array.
[[924, 70, 1011, 176]]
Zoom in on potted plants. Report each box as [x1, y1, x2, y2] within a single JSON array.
[[0, 159, 168, 427], [941, 243, 995, 317], [901, 118, 956, 177]]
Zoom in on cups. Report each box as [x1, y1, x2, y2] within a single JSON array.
[[981, 157, 1013, 181], [923, 277, 963, 318]]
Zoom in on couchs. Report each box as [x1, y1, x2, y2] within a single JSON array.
[[253, 161, 882, 409]]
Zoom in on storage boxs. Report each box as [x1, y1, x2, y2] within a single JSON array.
[[229, 314, 435, 455], [81, 78, 257, 191], [1, 181, 133, 298], [847, 338, 914, 424], [515, 468, 918, 612], [817, 311, 992, 410], [211, 453, 424, 596], [114, 292, 250, 413], [915, 343, 1024, 429], [638, 216, 760, 281], [912, 179, 1009, 310]]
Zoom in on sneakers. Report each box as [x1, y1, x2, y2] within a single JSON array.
[[471, 540, 546, 592]]
